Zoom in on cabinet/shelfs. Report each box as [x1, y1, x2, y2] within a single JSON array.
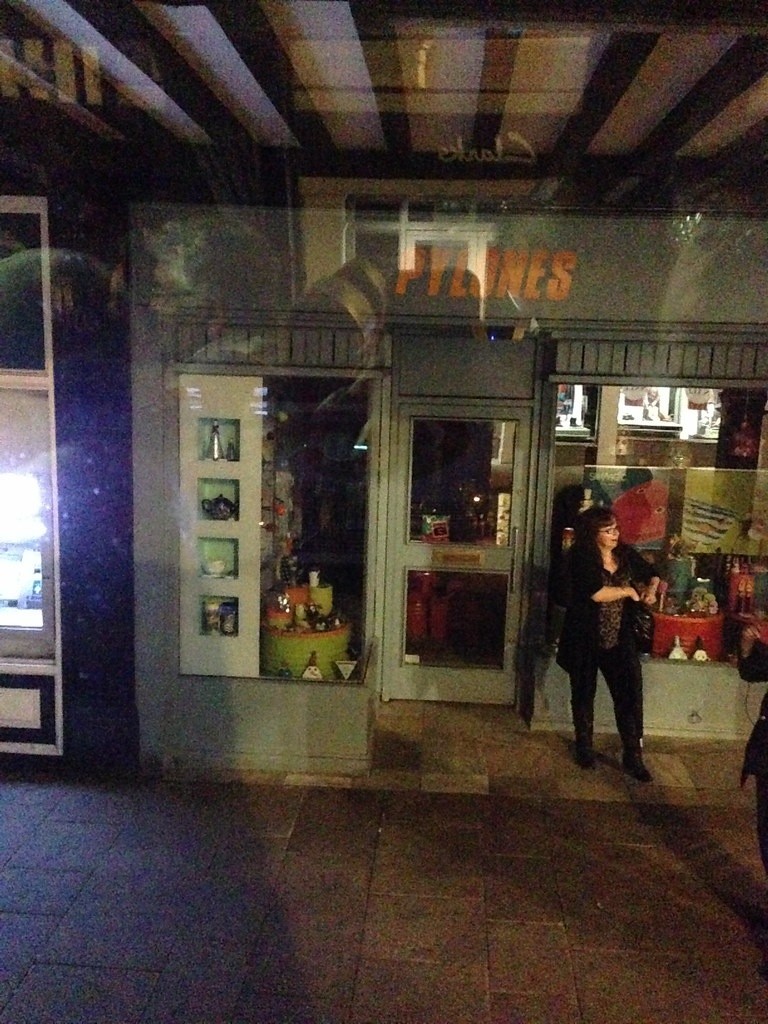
[[179, 373, 264, 679]]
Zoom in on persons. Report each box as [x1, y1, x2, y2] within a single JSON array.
[[638, 540, 695, 616], [555, 507, 660, 782], [738, 617, 768, 979]]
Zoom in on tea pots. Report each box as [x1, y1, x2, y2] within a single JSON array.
[[200, 493, 239, 520]]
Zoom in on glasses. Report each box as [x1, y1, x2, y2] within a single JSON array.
[[597, 528, 615, 534]]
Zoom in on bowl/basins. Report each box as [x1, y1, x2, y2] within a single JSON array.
[[200, 559, 227, 575]]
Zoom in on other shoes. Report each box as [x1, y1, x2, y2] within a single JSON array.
[[576, 742, 595, 770], [623, 752, 653, 782]]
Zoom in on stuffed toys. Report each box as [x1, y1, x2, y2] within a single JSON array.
[[685, 586, 718, 618]]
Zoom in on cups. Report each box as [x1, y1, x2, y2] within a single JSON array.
[[221, 602, 238, 634], [309, 583, 333, 615], [200, 600, 219, 635]]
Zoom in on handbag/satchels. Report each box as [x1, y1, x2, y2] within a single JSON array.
[[619, 545, 654, 653]]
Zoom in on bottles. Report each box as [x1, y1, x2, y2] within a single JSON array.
[[736, 588, 752, 618], [224, 437, 238, 460], [206, 418, 223, 460]]
